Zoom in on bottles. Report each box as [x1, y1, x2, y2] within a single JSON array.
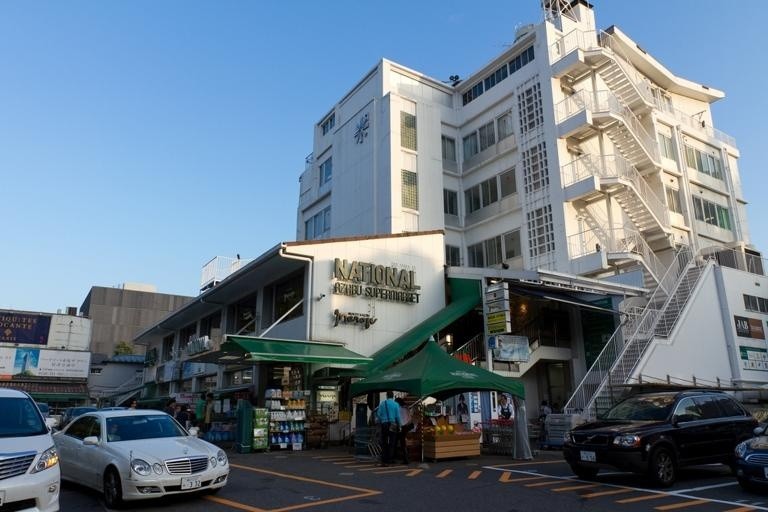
[[265, 388, 306, 432]]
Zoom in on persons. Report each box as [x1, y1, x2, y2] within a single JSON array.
[[377, 391, 414, 465], [107, 423, 121, 442], [158, 392, 214, 434], [457, 394, 468, 415], [539, 400, 568, 441], [128, 398, 137, 410], [498, 394, 515, 420]]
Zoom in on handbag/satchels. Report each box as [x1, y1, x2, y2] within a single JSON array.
[[388, 422, 397, 432]]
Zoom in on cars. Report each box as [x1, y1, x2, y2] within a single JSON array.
[[562, 389, 768, 494], [0, 388, 230, 512]]
